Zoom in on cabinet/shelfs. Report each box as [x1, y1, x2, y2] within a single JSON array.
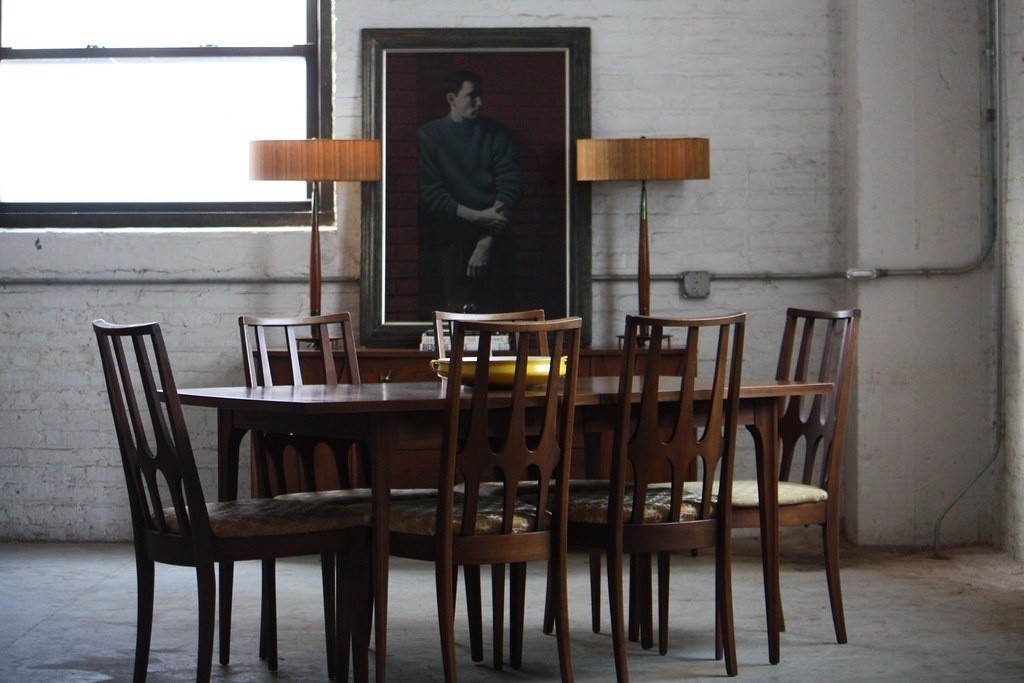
[[250, 346, 699, 556]]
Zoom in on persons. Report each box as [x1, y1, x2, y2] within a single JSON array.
[[418, 69, 523, 312]]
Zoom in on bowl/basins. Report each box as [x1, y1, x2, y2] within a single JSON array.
[[428, 356, 569, 390]]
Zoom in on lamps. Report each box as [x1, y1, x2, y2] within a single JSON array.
[[248, 137, 382, 350], [576, 135, 711, 349]]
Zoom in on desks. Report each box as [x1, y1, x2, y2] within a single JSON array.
[[155, 373, 836, 683]]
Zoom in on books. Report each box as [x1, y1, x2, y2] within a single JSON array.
[[418, 330, 510, 353]]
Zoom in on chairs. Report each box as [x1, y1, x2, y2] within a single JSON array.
[[92, 305, 863, 683]]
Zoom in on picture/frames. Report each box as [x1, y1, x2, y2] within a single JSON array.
[[358, 27, 592, 349]]
